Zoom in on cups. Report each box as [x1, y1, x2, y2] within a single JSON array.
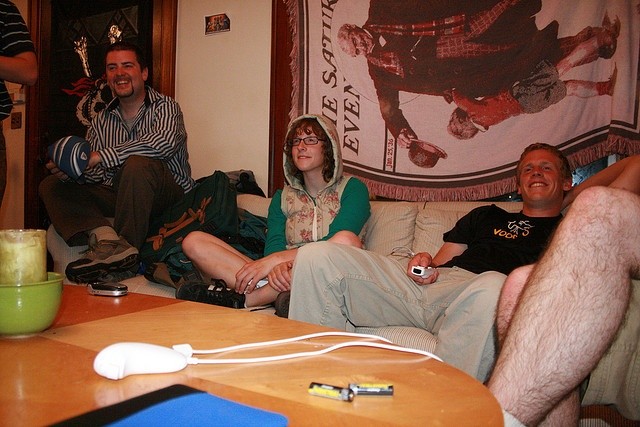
[[1, 230, 49, 284]]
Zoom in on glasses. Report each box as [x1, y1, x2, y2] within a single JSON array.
[[286, 136, 327, 146]]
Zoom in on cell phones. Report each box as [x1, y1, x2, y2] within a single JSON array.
[[88, 279, 128, 295]]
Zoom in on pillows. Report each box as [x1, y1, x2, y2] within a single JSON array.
[[412, 210, 468, 259], [356, 200, 416, 262]]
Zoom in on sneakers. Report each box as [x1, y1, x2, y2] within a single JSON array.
[[274, 290, 291, 318], [605, 62, 617, 96], [65, 233, 139, 285], [174, 279, 245, 310], [601, 9, 614, 45], [599, 16, 621, 58]]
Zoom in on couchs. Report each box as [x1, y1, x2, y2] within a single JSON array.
[[43, 187, 640, 426]]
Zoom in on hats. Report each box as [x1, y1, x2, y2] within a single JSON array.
[[47, 135, 90, 185], [408, 137, 448, 169]]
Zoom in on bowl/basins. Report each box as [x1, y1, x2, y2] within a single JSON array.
[[0, 273, 65, 340]]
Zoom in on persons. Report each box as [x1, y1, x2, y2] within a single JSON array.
[[448, 16, 621, 138], [176, 114, 370, 317], [487, 186, 640, 425], [288, 143, 639, 377], [39, 43, 194, 283], [1, 0, 38, 206], [337, 0, 618, 148]]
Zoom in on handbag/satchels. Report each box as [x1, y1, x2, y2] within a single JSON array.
[[138, 170, 238, 258]]
